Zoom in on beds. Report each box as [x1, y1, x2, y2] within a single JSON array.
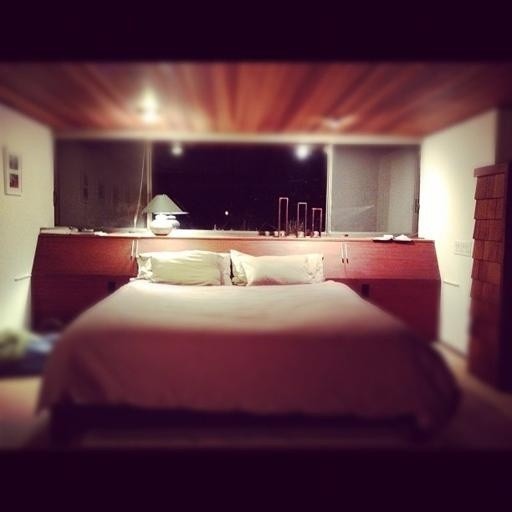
[[34, 279, 417, 413]]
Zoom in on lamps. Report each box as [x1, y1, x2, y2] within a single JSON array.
[[142, 195, 183, 235]]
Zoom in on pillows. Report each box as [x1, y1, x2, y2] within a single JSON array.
[[137, 250, 325, 287]]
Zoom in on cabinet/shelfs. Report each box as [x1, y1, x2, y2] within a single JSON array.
[[32, 233, 440, 340]]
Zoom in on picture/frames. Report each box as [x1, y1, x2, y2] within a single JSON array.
[[4, 144, 22, 195]]
